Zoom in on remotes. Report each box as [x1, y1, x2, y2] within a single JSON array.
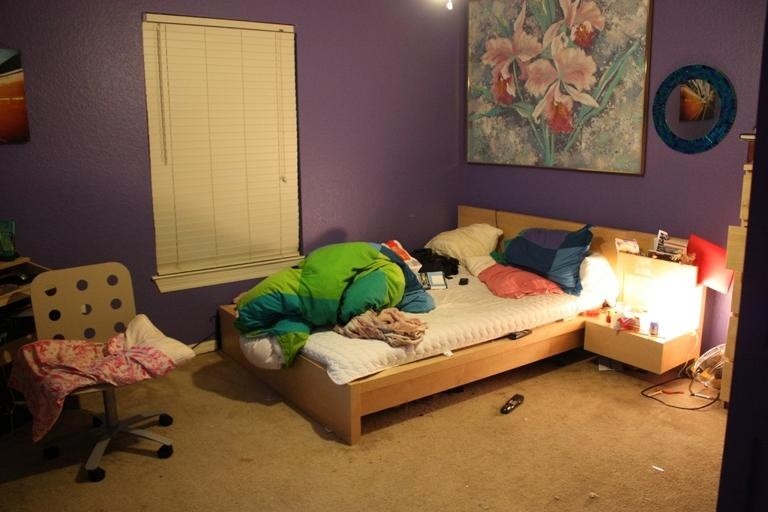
[[507, 329, 532, 341], [500, 393, 525, 414]]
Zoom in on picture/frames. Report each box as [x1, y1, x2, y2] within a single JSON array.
[[459, 0, 654, 177]]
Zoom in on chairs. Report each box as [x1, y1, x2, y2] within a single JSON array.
[[29, 260, 175, 485]]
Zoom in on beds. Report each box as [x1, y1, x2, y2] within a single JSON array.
[[217, 203, 658, 447]]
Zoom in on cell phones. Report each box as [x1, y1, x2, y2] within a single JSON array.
[[459, 276, 469, 285]]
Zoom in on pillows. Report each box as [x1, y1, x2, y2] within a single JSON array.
[[478, 262, 564, 299], [504, 224, 593, 295]]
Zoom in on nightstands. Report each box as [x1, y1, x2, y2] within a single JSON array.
[[583, 252, 707, 375]]
